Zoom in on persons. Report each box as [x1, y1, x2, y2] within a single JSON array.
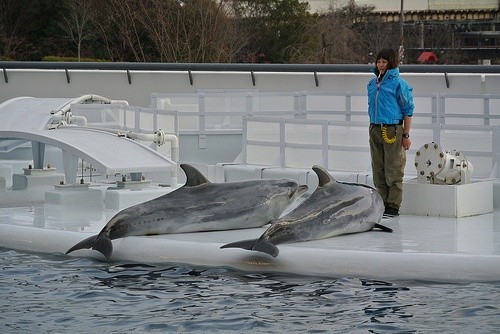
[[366, 47, 414, 218]]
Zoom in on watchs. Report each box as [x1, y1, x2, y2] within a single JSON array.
[[402, 132, 409, 138]]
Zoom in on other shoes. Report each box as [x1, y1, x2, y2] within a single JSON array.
[[383, 206, 399, 218]]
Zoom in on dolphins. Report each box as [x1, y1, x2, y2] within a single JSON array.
[[217, 166, 395, 258], [65, 163, 309, 261]]
